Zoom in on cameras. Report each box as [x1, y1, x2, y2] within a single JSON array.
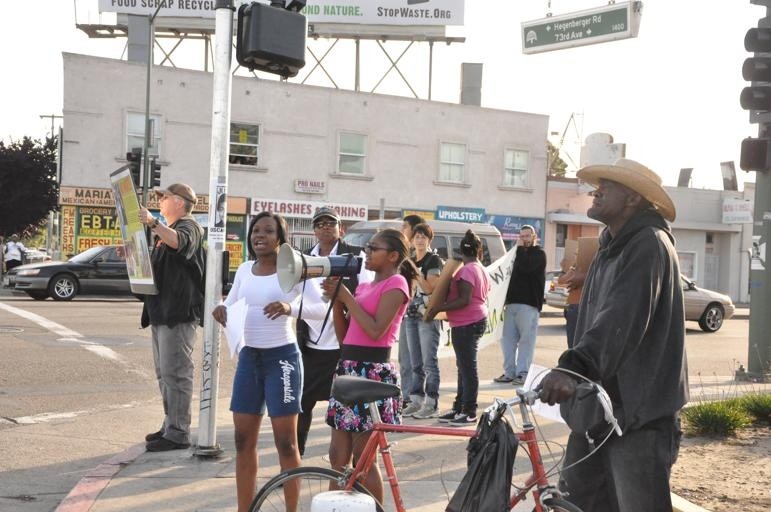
[[407, 295, 423, 318]]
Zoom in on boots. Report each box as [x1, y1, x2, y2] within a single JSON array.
[[402, 394, 440, 418]]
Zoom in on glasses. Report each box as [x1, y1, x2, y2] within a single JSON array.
[[363, 244, 390, 253], [314, 220, 338, 228]]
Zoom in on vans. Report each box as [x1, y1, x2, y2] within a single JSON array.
[[341, 219, 506, 269]]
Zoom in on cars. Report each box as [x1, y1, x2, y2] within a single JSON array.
[[546, 271, 736, 332], [3, 245, 154, 300]]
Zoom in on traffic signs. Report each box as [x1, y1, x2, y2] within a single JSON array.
[[522, 2, 640, 56]]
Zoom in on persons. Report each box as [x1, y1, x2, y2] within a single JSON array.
[[214, 210, 303, 512], [328, 229, 409, 512], [3, 234, 25, 285], [398, 222, 443, 420], [531, 157, 690, 512], [497, 227, 547, 384], [557, 267, 587, 349], [439, 229, 487, 427], [277, 206, 366, 488], [400, 214, 435, 266], [118, 182, 207, 450]]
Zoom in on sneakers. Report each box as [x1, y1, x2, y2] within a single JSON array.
[[145, 431, 190, 452], [437, 410, 479, 426], [494, 374, 524, 385]]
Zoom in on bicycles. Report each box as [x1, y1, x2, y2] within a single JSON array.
[[246, 375, 598, 512]]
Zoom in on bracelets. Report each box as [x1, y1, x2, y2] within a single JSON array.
[[151, 217, 160, 230]]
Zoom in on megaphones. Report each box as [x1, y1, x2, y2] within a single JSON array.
[[276, 243, 363, 293]]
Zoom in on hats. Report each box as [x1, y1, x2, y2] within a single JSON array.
[[151, 183, 198, 205], [313, 207, 341, 222], [576, 158, 676, 224]]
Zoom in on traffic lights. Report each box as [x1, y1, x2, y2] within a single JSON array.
[[152, 162, 161, 186], [126, 151, 140, 185], [739, 25, 770, 173]]
